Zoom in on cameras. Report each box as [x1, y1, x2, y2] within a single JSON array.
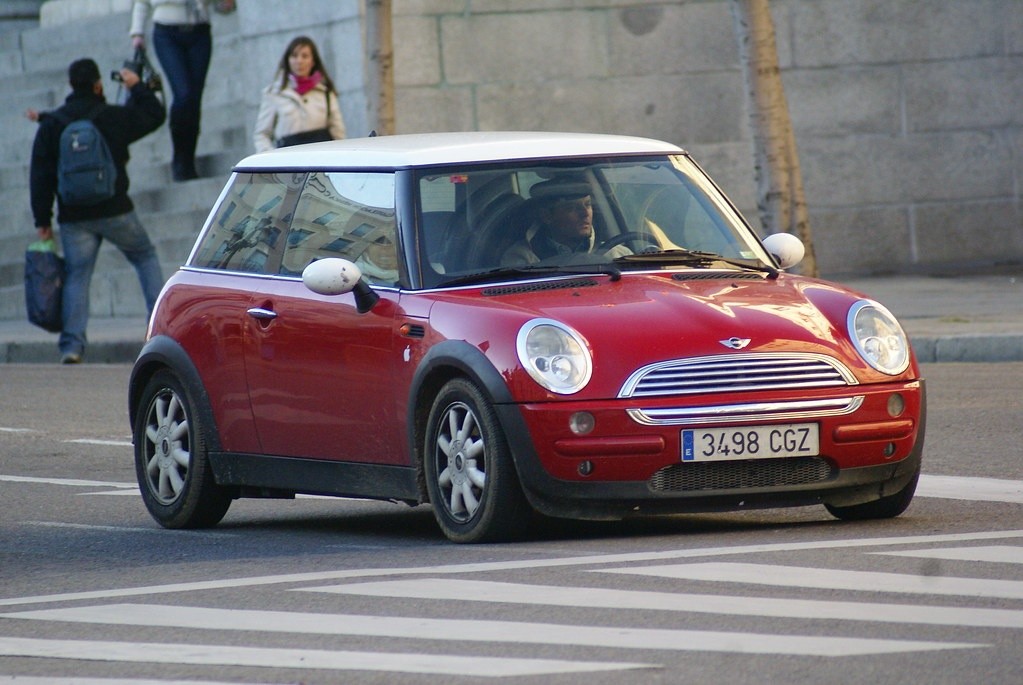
[[111, 72, 124, 81]]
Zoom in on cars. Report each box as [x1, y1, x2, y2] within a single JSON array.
[[128, 132, 927, 545]]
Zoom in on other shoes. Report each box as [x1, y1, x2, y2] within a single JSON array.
[[61, 353, 81, 362]]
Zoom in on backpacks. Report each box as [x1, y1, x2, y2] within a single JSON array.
[[49, 102, 117, 211]]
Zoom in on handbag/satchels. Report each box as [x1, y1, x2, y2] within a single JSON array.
[[115, 44, 164, 107], [24, 232, 67, 332]]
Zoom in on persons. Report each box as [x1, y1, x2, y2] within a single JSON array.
[[252, 37, 346, 154], [353, 205, 445, 290], [129, 0, 236, 182], [500, 194, 635, 271], [24, 59, 165, 363]]
